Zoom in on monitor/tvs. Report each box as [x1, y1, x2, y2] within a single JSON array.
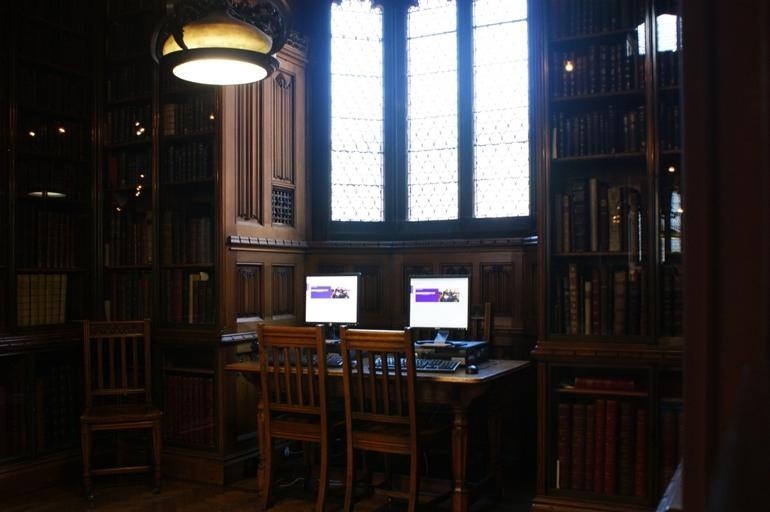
[[406, 273, 472, 348], [303, 272, 362, 341]]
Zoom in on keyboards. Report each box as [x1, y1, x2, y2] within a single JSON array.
[[268, 352, 343, 367], [368, 357, 461, 373]]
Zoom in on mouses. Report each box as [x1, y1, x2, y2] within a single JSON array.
[[466, 365, 478, 374], [350, 360, 357, 369]]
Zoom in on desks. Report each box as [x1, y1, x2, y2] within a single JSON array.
[[224, 357, 533, 511]]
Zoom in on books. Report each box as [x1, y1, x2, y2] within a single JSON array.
[[159, 138, 213, 263], [554, 174, 680, 253], [2, 365, 217, 458], [3, 3, 89, 327], [159, 2, 215, 135], [550, 0, 679, 36], [552, 261, 679, 337], [552, 37, 678, 97], [103, 149, 153, 323], [108, 2, 152, 145], [162, 271, 215, 324], [551, 101, 679, 157], [556, 375, 682, 499]]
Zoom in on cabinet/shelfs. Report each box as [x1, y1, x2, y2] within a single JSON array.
[[0, 0, 308, 485], [527, 2, 718, 512]]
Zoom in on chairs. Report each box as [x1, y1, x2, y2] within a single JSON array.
[[76, 311, 164, 500], [250, 321, 451, 512]]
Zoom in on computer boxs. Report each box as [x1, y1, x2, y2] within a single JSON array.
[[413, 339, 489, 366], [303, 341, 361, 360]]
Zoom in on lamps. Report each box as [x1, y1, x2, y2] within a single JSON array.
[[150, 0, 288, 85]]
